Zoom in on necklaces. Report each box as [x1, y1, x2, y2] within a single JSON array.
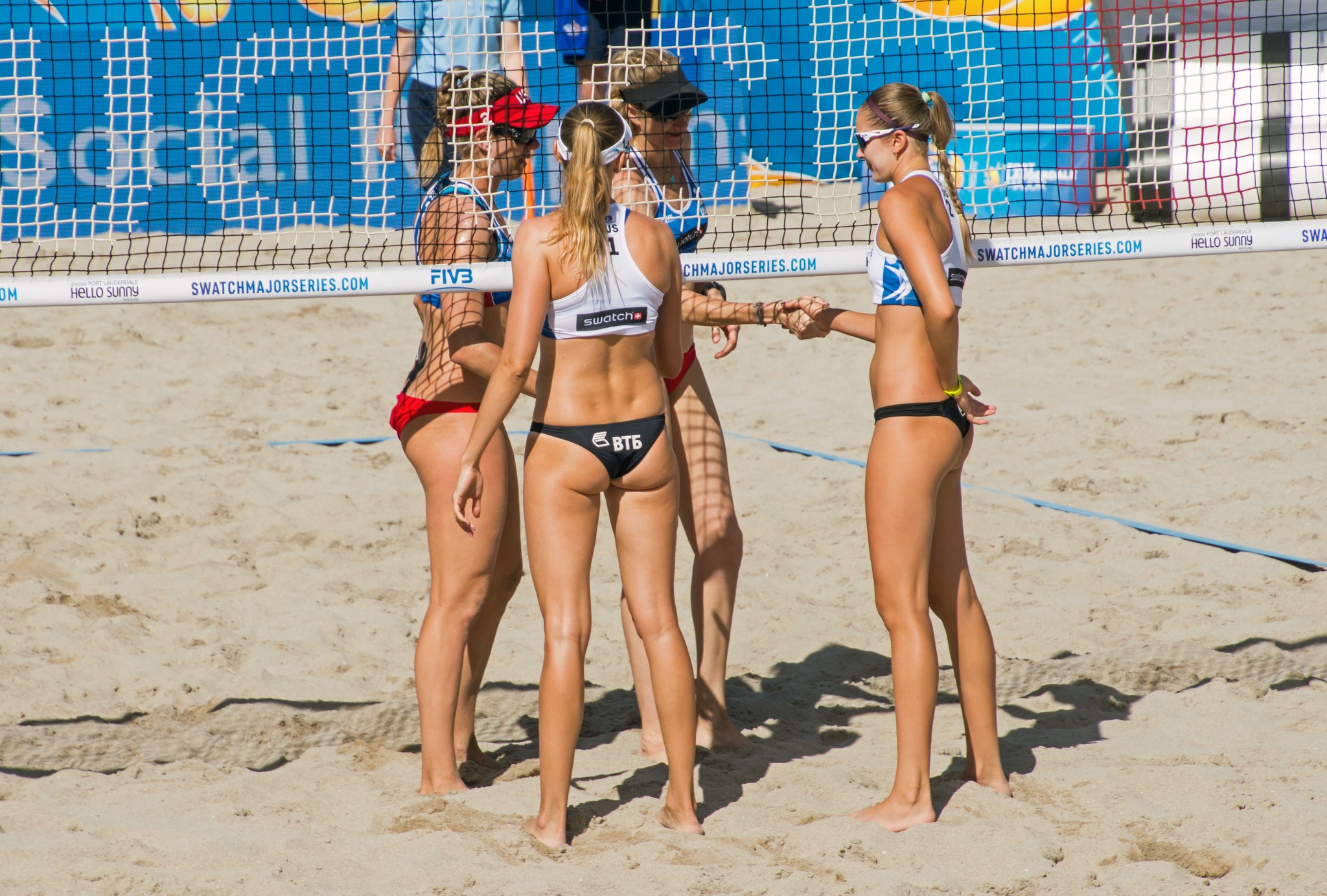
[[633, 139, 676, 183]]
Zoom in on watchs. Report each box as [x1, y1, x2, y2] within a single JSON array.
[[701, 281, 726, 301]]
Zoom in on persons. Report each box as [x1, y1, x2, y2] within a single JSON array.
[[387, 65, 562, 796], [568, 0, 653, 101], [377, 0, 528, 193], [607, 47, 829, 764], [782, 82, 1013, 833], [453, 100, 705, 851]]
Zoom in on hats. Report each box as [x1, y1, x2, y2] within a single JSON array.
[[440, 85, 561, 139], [557, 107, 632, 166], [617, 67, 709, 116]]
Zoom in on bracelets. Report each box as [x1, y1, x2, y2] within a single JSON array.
[[944, 373, 963, 396], [752, 302, 766, 326]]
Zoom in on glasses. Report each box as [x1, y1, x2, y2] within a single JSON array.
[[486, 127, 538, 145], [853, 123, 920, 149], [650, 108, 694, 123], [554, 133, 631, 155]]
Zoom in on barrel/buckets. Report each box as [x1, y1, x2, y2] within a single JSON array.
[[1128, 120, 1290, 221]]
